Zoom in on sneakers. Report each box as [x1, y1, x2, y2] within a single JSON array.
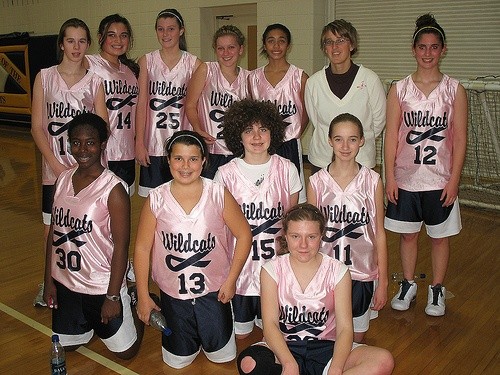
[[33, 282, 47, 307], [391, 279, 418, 311], [127, 286, 159, 305], [126, 259, 136, 282], [424, 284, 446, 316]]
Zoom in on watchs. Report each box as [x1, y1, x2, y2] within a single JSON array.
[[106, 293, 121, 301]]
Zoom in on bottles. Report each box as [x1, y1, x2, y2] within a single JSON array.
[[392, 271, 425, 282], [149, 309, 172, 336], [49, 335, 67, 375]]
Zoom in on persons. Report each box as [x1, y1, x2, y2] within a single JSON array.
[[246, 23, 310, 206], [184, 25, 251, 181], [30, 18, 111, 308], [236, 203, 395, 375], [384, 13, 469, 316], [306, 113, 390, 345], [43, 113, 144, 360], [303, 18, 387, 174], [82, 13, 140, 284], [133, 130, 252, 369], [212, 97, 303, 340], [134, 7, 204, 201]]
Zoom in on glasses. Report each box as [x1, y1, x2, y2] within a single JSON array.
[[323, 37, 350, 46]]
